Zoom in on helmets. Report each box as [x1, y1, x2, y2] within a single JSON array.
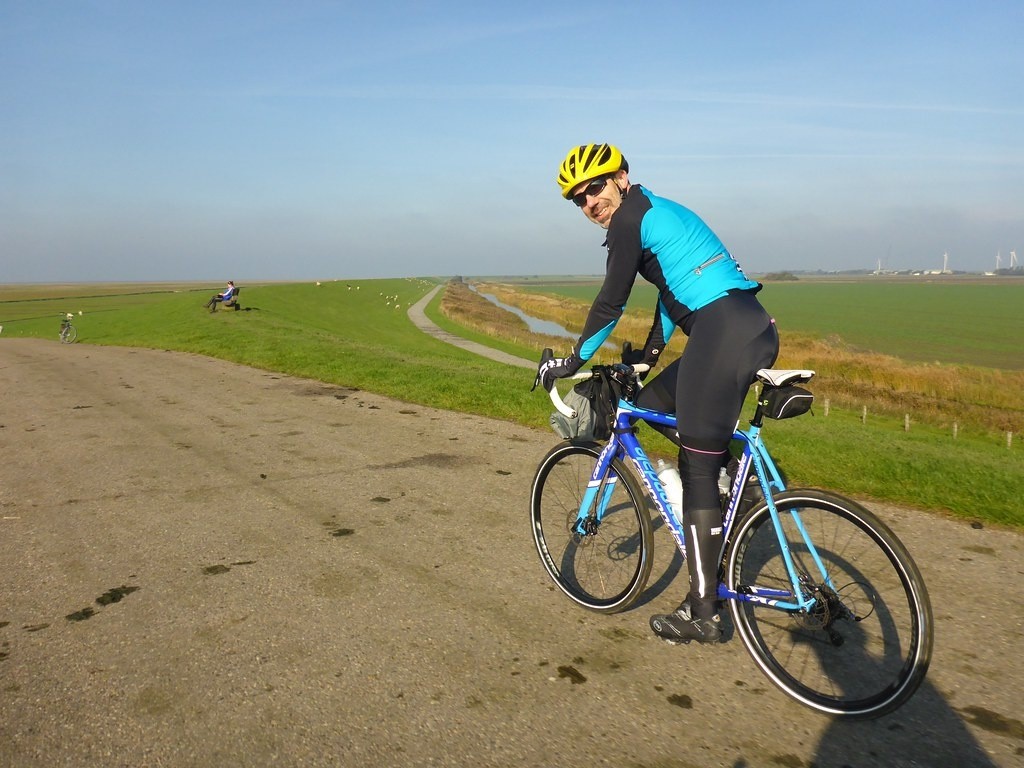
[[556, 141, 630, 201]]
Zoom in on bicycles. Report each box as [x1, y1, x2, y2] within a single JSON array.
[[58, 311, 83, 343], [529, 346, 937, 720]]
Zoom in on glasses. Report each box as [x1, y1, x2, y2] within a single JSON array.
[[572, 173, 614, 207]]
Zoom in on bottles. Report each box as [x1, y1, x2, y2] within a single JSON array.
[[655, 458, 684, 525], [716, 467, 731, 500]]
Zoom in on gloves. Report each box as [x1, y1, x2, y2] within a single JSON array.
[[538, 346, 587, 393], [636, 343, 661, 381]]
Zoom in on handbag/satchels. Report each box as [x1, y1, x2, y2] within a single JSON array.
[[548, 364, 626, 442]]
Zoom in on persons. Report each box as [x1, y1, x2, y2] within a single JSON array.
[[538, 144, 780, 648], [203, 281, 236, 313]]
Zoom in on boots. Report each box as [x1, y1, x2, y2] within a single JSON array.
[[718, 455, 765, 526], [649, 507, 724, 645]]
